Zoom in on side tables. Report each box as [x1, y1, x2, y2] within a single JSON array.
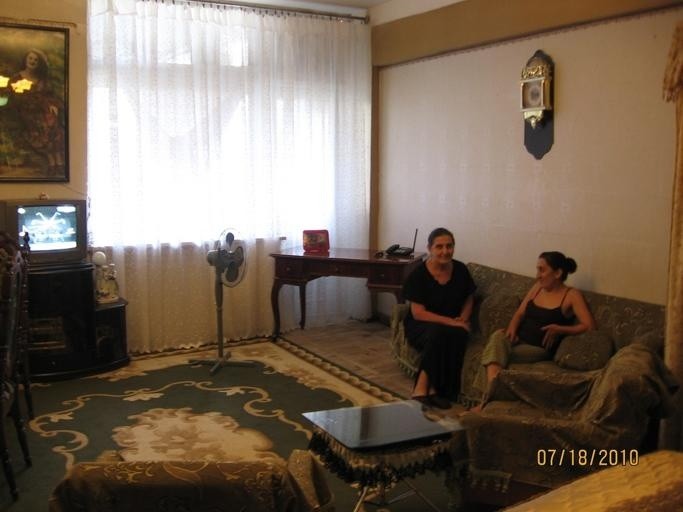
[[297, 396, 469, 511]]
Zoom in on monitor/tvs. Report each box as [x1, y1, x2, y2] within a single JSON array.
[[0, 197, 88, 269]]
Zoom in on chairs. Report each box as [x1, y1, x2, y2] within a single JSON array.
[[456, 334, 663, 511], [0, 232, 33, 504], [46, 447, 332, 509]]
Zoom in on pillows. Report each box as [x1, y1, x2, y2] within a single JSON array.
[[553, 330, 615, 371], [479, 293, 521, 340]]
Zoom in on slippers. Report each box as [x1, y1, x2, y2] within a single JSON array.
[[412, 391, 452, 410]]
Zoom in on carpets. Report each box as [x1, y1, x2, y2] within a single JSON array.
[[2, 333, 461, 512]]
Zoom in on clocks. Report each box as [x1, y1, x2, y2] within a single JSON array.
[[519, 56, 554, 131]]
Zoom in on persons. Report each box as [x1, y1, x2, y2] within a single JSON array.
[[458, 251, 596, 417], [7, 50, 65, 172], [404, 227, 479, 408]]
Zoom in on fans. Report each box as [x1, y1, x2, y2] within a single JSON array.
[[188, 232, 255, 376]]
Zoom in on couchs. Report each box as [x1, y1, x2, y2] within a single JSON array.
[[388, 261, 666, 411]]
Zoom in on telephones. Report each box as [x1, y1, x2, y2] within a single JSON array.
[[385, 244, 412, 258]]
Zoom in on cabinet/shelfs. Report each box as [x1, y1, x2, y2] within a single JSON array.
[[6, 260, 129, 383]]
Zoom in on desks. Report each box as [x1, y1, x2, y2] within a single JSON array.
[[268, 245, 428, 341]]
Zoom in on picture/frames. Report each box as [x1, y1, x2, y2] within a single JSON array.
[[0, 20, 71, 182]]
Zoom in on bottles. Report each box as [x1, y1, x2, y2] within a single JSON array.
[[100, 262, 116, 297]]
